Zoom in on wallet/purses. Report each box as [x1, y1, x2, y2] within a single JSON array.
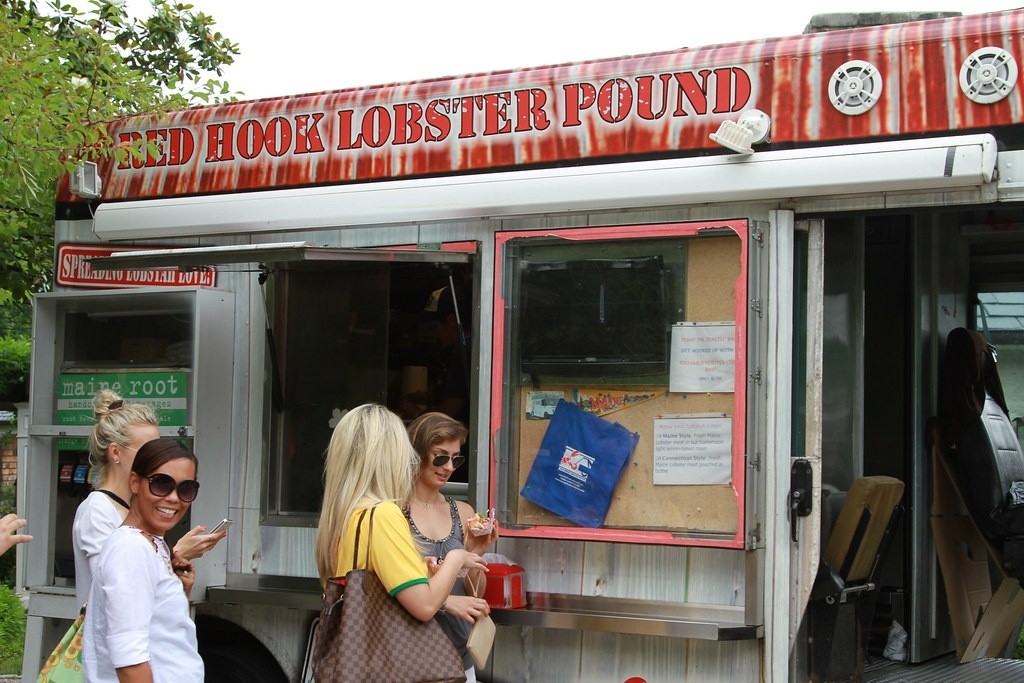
[[465, 571, 497, 671]]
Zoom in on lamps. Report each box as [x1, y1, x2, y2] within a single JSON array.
[[66, 155, 102, 200], [709, 108, 773, 156]]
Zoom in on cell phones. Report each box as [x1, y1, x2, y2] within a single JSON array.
[[209, 518, 234, 534]]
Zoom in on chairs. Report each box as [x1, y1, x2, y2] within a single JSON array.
[[813, 475, 904, 606], [932, 319, 1024, 584]]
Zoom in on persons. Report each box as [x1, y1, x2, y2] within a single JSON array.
[[311, 403, 489, 683], [406, 411, 499, 683], [71, 389, 234, 616], [81, 438, 205, 683], [0, 512, 34, 556]]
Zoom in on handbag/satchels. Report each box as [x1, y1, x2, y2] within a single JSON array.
[[520, 398, 640, 528], [35, 600, 88, 683], [312, 500, 468, 683]]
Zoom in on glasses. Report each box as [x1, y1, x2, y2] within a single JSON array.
[[145, 473, 200, 503], [429, 456, 466, 469]]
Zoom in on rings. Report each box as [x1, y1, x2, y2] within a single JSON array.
[[208, 534, 212, 541], [183, 570, 188, 577]]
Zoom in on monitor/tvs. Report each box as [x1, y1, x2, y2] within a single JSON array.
[[520, 255, 668, 379]]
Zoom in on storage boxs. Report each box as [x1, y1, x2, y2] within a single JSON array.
[[485, 563, 529, 610]]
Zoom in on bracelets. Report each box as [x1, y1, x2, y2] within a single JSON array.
[[437, 601, 446, 615]]
